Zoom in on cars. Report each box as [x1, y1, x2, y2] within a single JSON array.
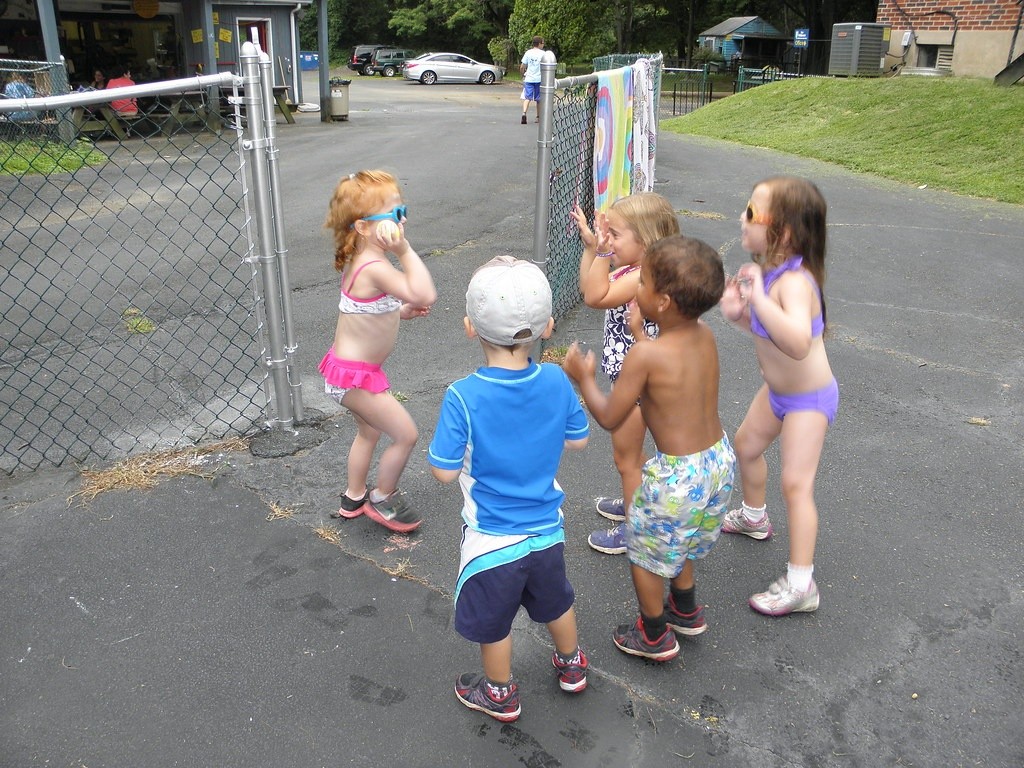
[[402, 52, 503, 85]]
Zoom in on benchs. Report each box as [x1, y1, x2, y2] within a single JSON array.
[[139, 109, 226, 118], [20, 118, 61, 124], [221, 102, 300, 115], [115, 115, 141, 120]]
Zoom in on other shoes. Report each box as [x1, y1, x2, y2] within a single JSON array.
[[534, 117, 539, 123], [520, 112, 528, 124]]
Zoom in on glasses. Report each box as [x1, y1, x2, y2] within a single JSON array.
[[745, 202, 773, 227], [348, 205, 407, 230]]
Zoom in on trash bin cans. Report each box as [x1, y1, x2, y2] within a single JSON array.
[[329, 77, 352, 121]]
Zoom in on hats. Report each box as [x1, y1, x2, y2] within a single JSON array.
[[466, 255, 553, 345]]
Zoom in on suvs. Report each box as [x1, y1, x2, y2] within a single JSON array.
[[348, 45, 404, 76], [371, 46, 427, 77]]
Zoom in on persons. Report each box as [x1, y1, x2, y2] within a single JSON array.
[[318, 169, 438, 531], [428, 254, 591, 721], [569, 192, 680, 554], [85, 63, 138, 137], [720, 177, 839, 616], [562, 235, 736, 661], [0, 71, 42, 141], [520, 36, 545, 124]]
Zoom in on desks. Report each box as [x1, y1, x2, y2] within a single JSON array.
[[744, 67, 805, 80], [220, 85, 295, 125], [69, 90, 128, 141], [146, 90, 208, 138]]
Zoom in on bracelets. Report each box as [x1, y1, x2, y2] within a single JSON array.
[[596, 252, 612, 257]]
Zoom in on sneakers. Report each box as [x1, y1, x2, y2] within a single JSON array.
[[612, 614, 680, 662], [339, 484, 377, 518], [663, 595, 708, 636], [364, 488, 422, 533], [588, 521, 628, 555], [748, 574, 821, 616], [454, 672, 522, 722], [595, 497, 627, 521], [719, 509, 773, 540], [552, 647, 588, 692]]
